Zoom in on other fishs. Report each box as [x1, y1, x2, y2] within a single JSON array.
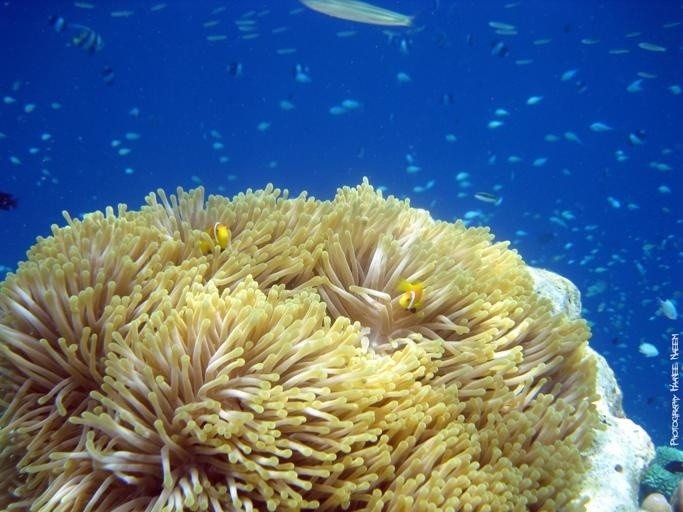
[[664, 461, 683, 474], [0, 0, 683, 447]]
